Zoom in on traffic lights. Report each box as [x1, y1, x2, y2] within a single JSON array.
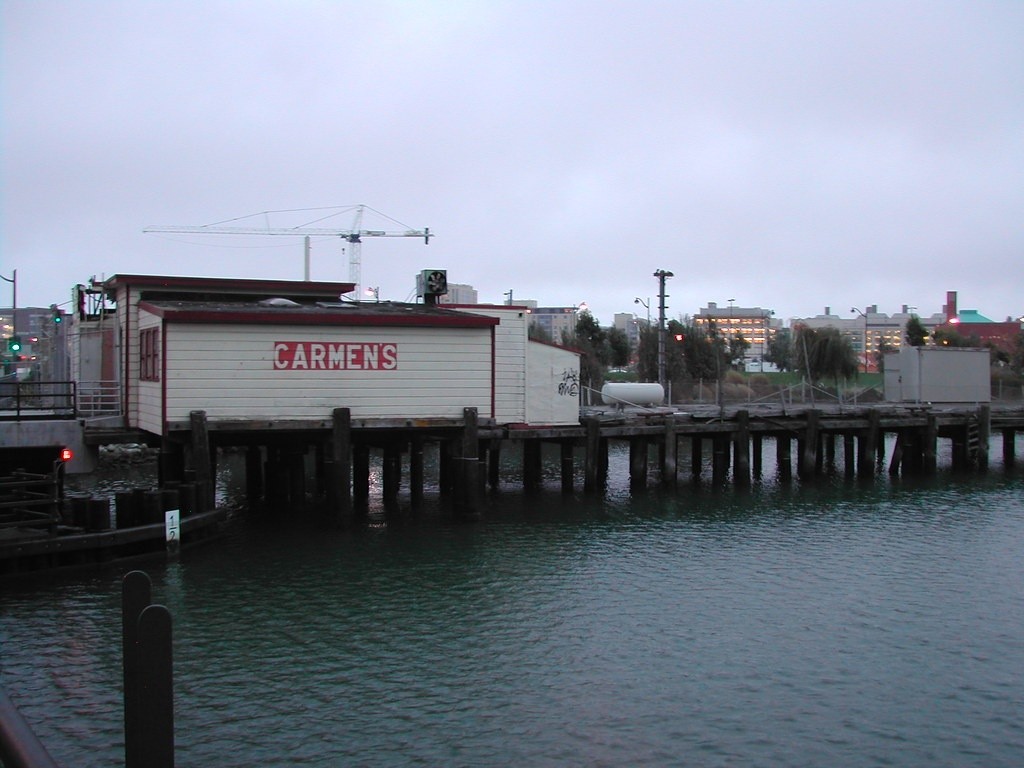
[[674, 334, 683, 343], [55, 309, 62, 324], [11, 341, 21, 352]]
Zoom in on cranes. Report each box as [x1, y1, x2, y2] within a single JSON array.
[[140, 201, 435, 302]]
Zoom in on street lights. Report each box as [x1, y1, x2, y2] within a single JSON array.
[[634, 296, 650, 382], [851, 306, 868, 375], [760, 309, 775, 373], [727, 299, 736, 340]]
